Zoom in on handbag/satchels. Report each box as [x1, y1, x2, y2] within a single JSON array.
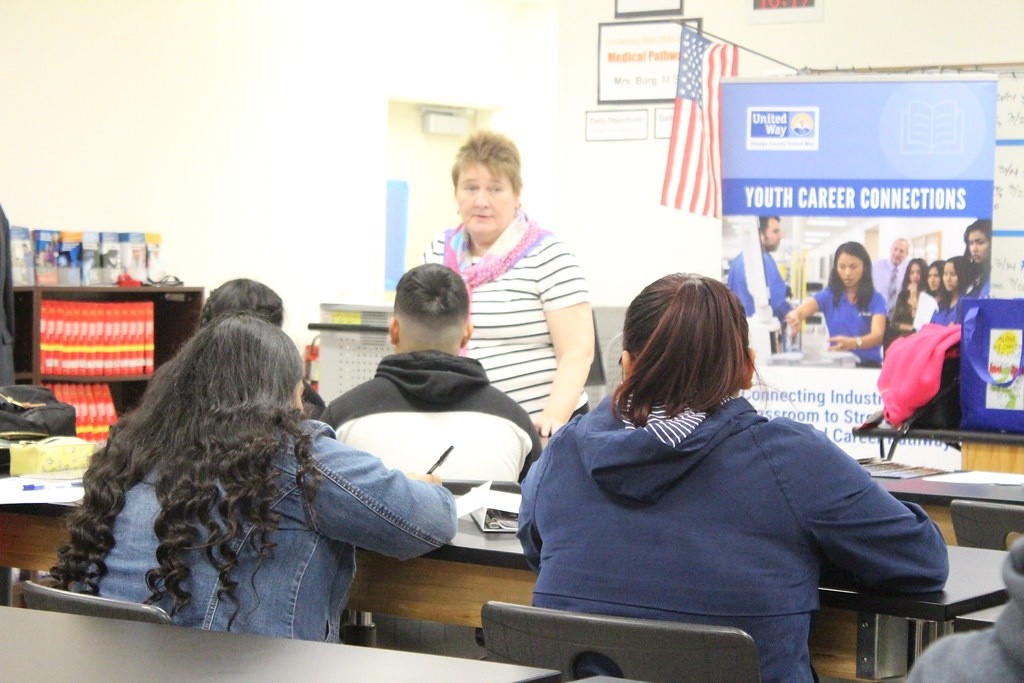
[[0, 384, 75, 439], [880, 357, 959, 461], [959, 300, 1024, 433]]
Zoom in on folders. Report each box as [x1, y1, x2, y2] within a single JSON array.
[[468, 487, 521, 534]]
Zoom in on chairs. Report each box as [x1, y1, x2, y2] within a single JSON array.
[[481, 601, 761, 683]]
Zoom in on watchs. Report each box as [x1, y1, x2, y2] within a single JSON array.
[[856, 338, 862, 350]]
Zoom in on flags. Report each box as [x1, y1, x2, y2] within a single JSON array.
[[661, 32, 738, 218]]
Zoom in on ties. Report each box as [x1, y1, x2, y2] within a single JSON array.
[[888, 270, 898, 316]]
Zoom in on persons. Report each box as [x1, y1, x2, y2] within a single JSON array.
[[196, 280, 324, 407], [53, 313, 457, 643], [729, 216, 991, 369], [907, 534, 1024, 683], [419, 133, 595, 440], [322, 263, 540, 483], [520, 274, 950, 683]]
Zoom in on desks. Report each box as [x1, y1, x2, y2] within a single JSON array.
[[0, 503, 1007, 683], [870, 470, 1024, 545], [0, 606, 562, 683]]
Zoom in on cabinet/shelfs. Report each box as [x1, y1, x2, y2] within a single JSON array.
[[12, 286, 205, 413]]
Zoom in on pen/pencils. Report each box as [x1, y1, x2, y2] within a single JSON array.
[[22, 482, 84, 490], [427, 445, 454, 475]]
